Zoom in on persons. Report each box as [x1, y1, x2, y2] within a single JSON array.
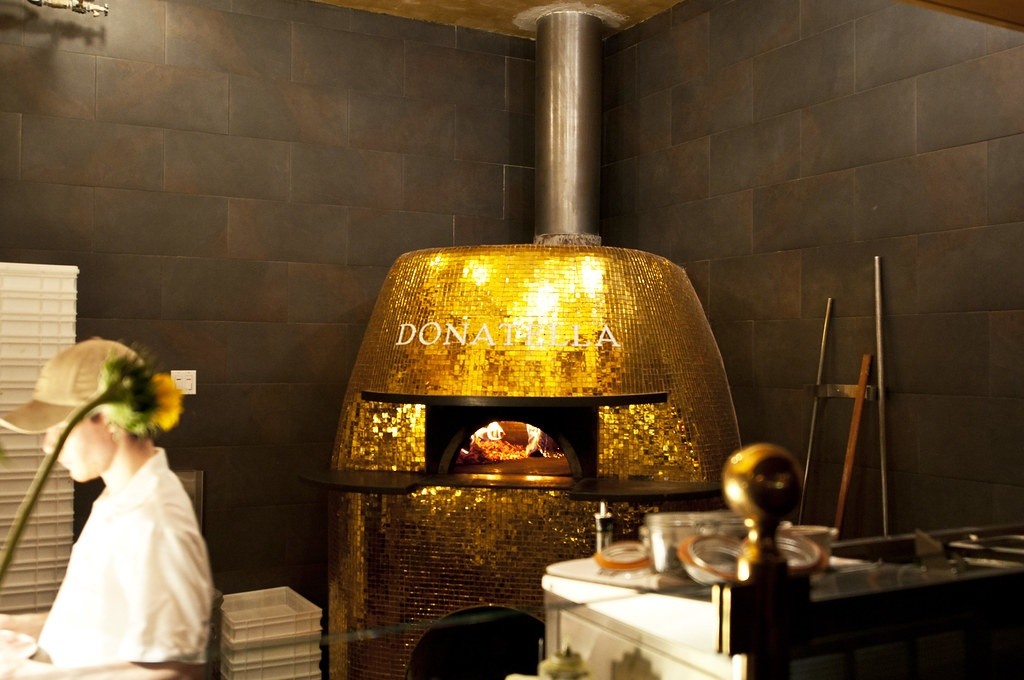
[[1, 335, 214, 680]]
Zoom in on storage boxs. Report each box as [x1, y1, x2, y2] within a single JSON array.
[[0, 262, 323, 680]]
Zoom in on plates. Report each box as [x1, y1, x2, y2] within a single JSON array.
[[0, 628, 39, 680]]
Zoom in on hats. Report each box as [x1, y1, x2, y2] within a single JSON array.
[[0, 337, 144, 434]]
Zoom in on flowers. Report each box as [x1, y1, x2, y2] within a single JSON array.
[[0, 337, 185, 586]]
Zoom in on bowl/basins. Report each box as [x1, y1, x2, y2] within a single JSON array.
[[644, 510, 750, 578], [780, 521, 838, 575]]
